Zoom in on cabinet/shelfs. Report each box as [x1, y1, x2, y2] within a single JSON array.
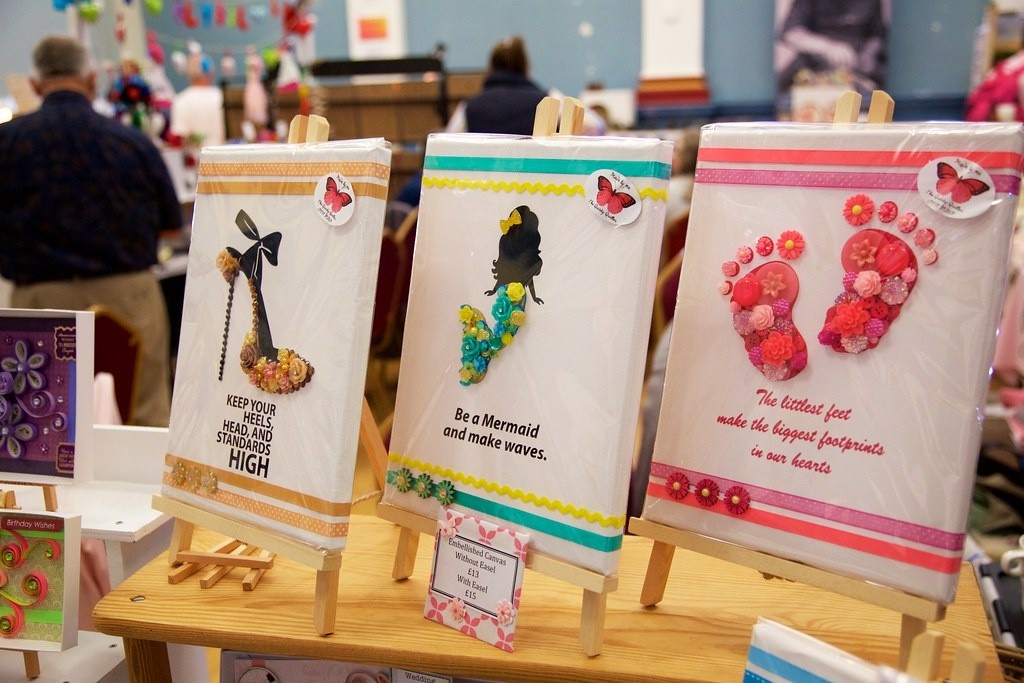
[[0, 424, 210, 683]]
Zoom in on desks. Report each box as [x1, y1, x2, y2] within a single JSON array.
[[92, 512, 1003, 683]]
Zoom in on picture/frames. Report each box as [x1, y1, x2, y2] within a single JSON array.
[[0, 308, 95, 486], [0, 509, 82, 652]]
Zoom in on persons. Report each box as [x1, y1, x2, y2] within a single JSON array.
[[666, 136, 700, 221], [778, 0, 888, 95], [0, 36, 185, 427], [968, 23, 1024, 125], [392, 140, 426, 208], [445, 39, 606, 136]]
